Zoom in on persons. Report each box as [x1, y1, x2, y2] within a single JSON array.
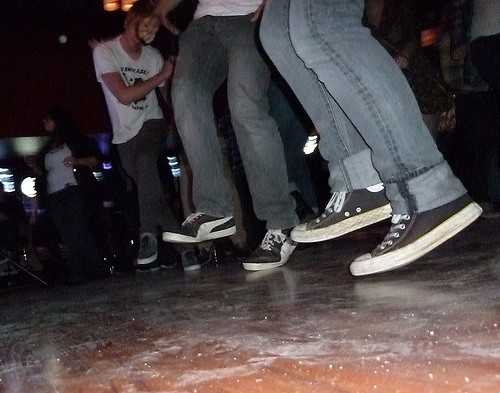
[[259, 0, 484, 275], [361, 0, 429, 103], [451, 0, 500, 218], [170, 80, 323, 265], [20, 107, 103, 284], [154, 0, 299, 270], [93, 1, 201, 270]]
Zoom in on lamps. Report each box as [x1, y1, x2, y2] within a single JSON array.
[[103, 0, 138, 12]]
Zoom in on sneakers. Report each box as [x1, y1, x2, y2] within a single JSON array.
[[162, 212, 236, 242], [243, 228, 299, 270], [290, 188, 391, 243], [137, 234, 158, 264], [350, 194, 482, 276], [181, 250, 200, 270]]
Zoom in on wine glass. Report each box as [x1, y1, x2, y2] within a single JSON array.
[[63, 157, 78, 174]]
